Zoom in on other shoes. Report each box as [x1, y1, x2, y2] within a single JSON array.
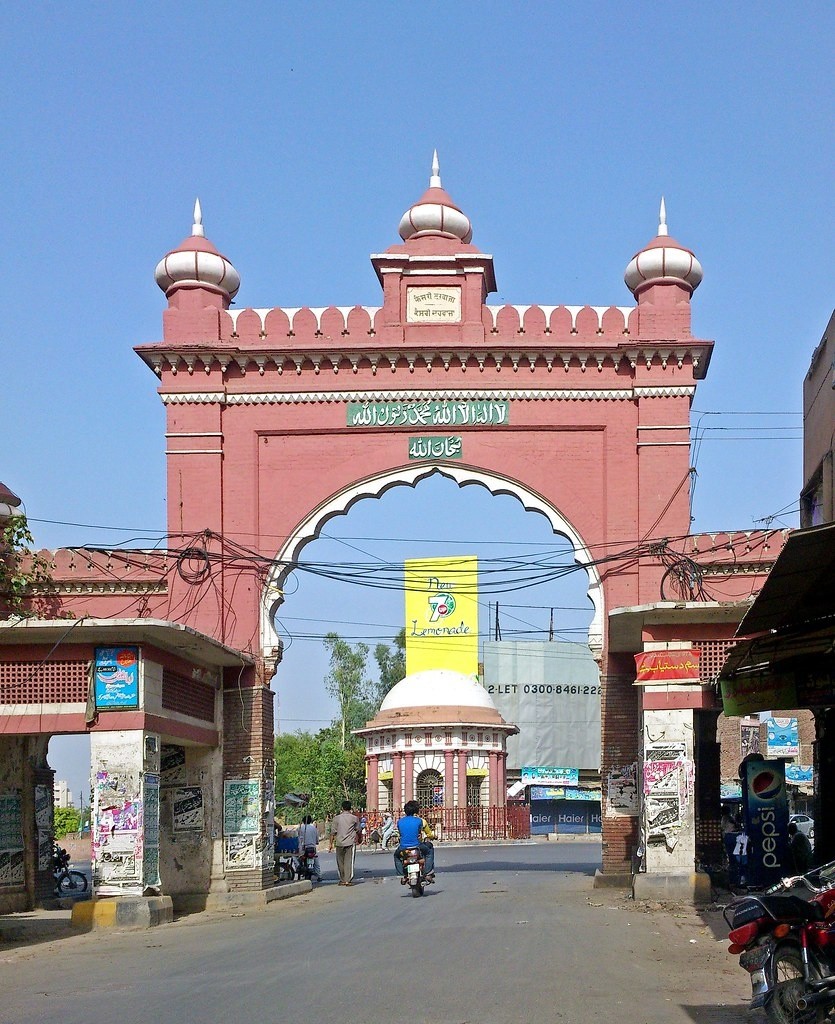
[[317, 877, 323, 882], [426, 875, 436, 884], [338, 880, 351, 886], [429, 873, 435, 877], [296, 865, 301, 874], [401, 876, 409, 885]]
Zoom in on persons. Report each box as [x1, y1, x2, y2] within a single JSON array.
[[292, 815, 323, 882], [381, 813, 394, 849], [790, 823, 812, 864], [394, 801, 435, 886], [721, 805, 738, 832], [274, 821, 282, 882], [328, 801, 361, 886]]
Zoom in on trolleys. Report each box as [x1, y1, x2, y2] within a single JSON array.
[[273, 853, 300, 881]]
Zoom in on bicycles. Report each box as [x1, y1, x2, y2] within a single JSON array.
[[361, 826, 399, 855]]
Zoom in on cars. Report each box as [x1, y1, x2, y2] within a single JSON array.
[[789, 814, 814, 838]]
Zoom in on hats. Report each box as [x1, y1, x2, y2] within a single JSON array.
[[384, 812, 392, 816]]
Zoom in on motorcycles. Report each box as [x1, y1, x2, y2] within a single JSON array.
[[723, 861, 835, 1024], [298, 847, 318, 881], [53, 845, 87, 893], [399, 837, 438, 897]]
